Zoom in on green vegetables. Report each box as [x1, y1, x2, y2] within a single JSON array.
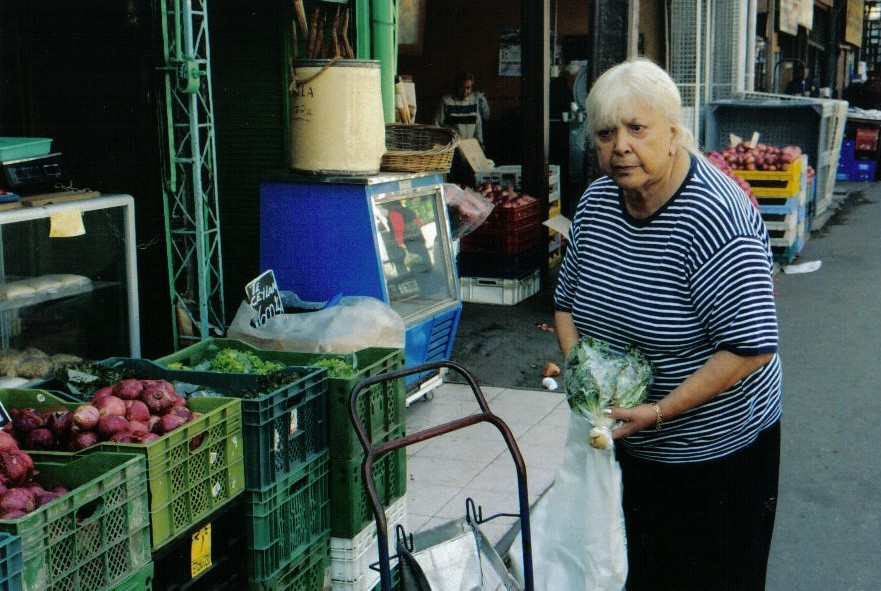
[[45, 360, 137, 402], [165, 348, 358, 398], [563, 338, 653, 449]]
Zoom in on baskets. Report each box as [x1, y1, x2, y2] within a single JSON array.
[[381, 122, 459, 171], [460, 183, 542, 252]]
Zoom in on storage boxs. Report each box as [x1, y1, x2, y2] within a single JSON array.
[[0, 339, 406, 591], [835, 129, 878, 183], [712, 154, 817, 262], [444, 162, 564, 307]]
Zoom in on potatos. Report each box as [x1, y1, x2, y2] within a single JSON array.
[[712, 140, 802, 172], [468, 182, 536, 208]]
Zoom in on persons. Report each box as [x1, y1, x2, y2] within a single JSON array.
[[784, 62, 820, 97], [554, 58, 781, 591], [431, 70, 490, 159], [863, 71, 876, 88], [383, 201, 432, 297]]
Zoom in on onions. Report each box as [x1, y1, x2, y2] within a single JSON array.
[[0, 379, 203, 521]]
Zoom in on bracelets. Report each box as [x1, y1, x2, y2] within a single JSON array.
[[650, 403, 662, 431]]
